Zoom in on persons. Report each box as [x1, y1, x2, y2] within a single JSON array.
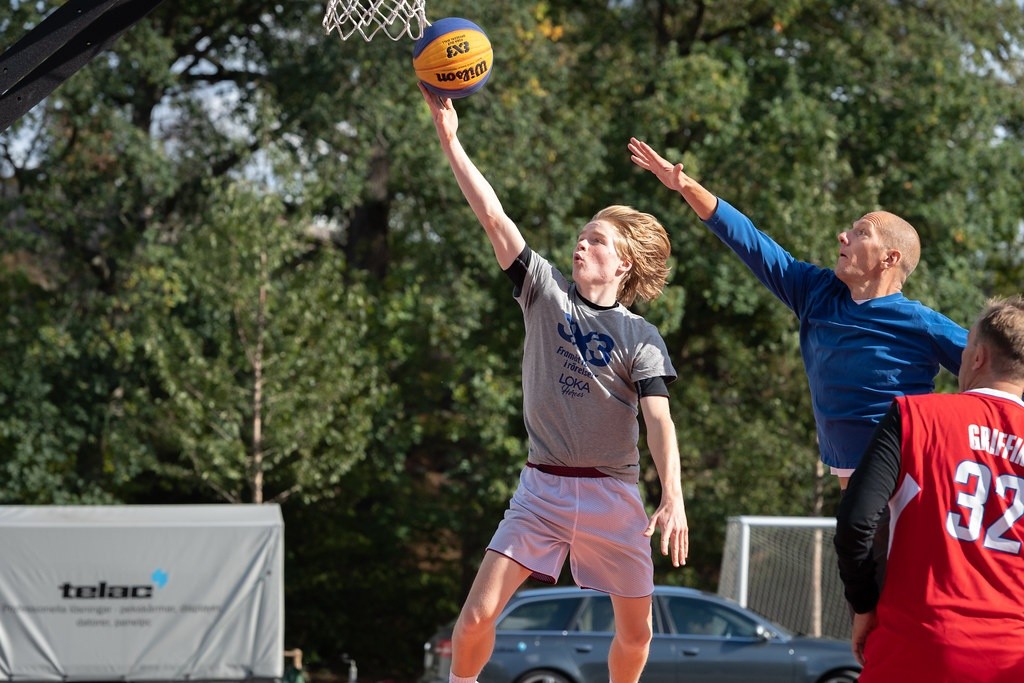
[[417, 82, 689, 683], [628, 137, 971, 626], [834, 294, 1024, 683]]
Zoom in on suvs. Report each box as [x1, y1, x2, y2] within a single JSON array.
[[420, 586, 863, 683]]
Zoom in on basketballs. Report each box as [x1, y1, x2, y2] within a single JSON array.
[[412, 16, 494, 99]]
